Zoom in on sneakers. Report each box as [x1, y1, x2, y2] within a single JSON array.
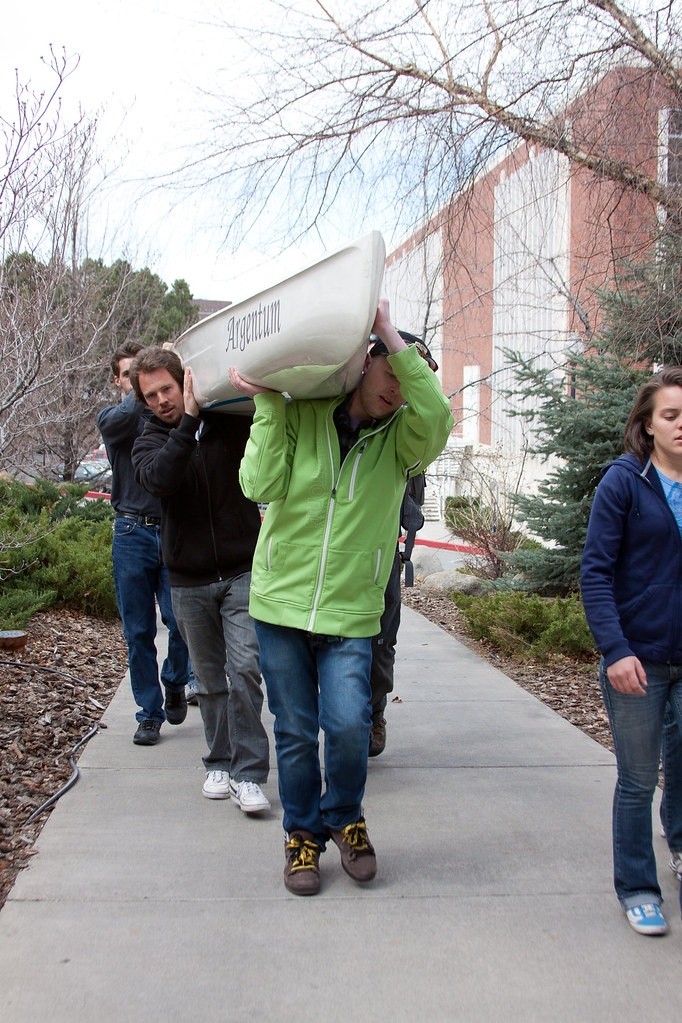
[[626, 903, 667, 935], [133, 720, 162, 745], [202, 770, 231, 799], [669, 853, 682, 881], [328, 806, 377, 881], [228, 777, 271, 811], [164, 686, 187, 725], [283, 831, 326, 895]]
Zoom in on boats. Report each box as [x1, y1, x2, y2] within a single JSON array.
[[172, 227, 386, 416]]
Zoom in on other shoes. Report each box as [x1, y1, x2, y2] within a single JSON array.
[[369, 709, 387, 756], [186, 692, 198, 706]]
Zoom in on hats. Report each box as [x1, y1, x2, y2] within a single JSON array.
[[370, 331, 432, 363]]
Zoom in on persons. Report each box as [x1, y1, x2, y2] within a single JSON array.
[[95, 343, 199, 746], [130, 345, 269, 811], [581, 367, 682, 933], [369, 491, 425, 756], [228, 299, 454, 896]]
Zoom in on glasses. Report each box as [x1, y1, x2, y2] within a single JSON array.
[[376, 337, 439, 372]]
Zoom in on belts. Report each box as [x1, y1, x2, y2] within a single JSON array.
[[115, 512, 161, 526]]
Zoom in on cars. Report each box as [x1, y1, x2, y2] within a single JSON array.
[[2, 436, 112, 493]]
